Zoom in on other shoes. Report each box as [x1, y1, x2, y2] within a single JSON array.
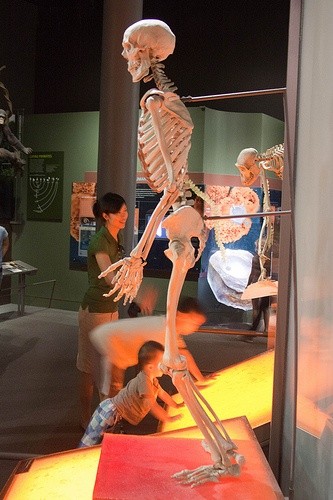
[[241, 337, 253, 343]]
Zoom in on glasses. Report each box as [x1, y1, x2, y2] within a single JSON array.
[[118, 244, 126, 256]]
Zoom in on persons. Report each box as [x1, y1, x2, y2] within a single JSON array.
[[0, 226, 9, 286], [87, 296, 220, 401], [76, 194, 154, 425], [239, 239, 270, 342], [79, 341, 185, 448]]
